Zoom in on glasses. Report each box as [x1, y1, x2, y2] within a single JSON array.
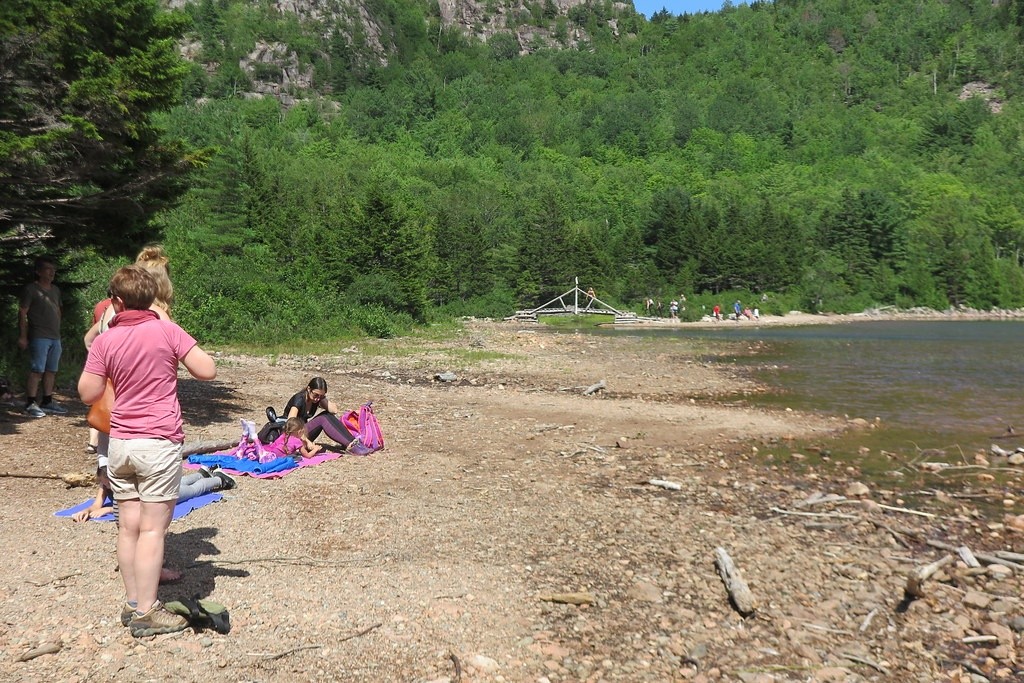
[[311, 390, 326, 400], [106, 290, 122, 300]]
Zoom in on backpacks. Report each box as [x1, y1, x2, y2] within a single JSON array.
[[256, 421, 286, 444], [339, 401, 384, 451], [165, 596, 230, 634]]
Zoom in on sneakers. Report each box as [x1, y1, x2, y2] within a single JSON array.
[[197, 466, 214, 478], [121, 600, 136, 627], [38, 400, 68, 413], [21, 400, 46, 418], [213, 468, 237, 489], [130, 599, 190, 637]]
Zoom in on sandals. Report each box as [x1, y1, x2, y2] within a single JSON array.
[[87, 444, 98, 454]]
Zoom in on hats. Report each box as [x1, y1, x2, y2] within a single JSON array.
[[738, 300, 741, 303]]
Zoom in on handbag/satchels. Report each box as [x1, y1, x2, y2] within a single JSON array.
[[85, 377, 115, 435]]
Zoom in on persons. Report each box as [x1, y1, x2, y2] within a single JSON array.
[[283, 377, 375, 455], [744, 306, 758, 321], [85, 246, 175, 483], [16, 262, 69, 418], [656, 300, 663, 319], [647, 298, 656, 317], [72, 453, 239, 523], [670, 298, 679, 318], [237, 417, 323, 464], [679, 294, 687, 312], [712, 304, 724, 321], [733, 300, 749, 320], [586, 287, 596, 310], [77, 264, 216, 637]]
